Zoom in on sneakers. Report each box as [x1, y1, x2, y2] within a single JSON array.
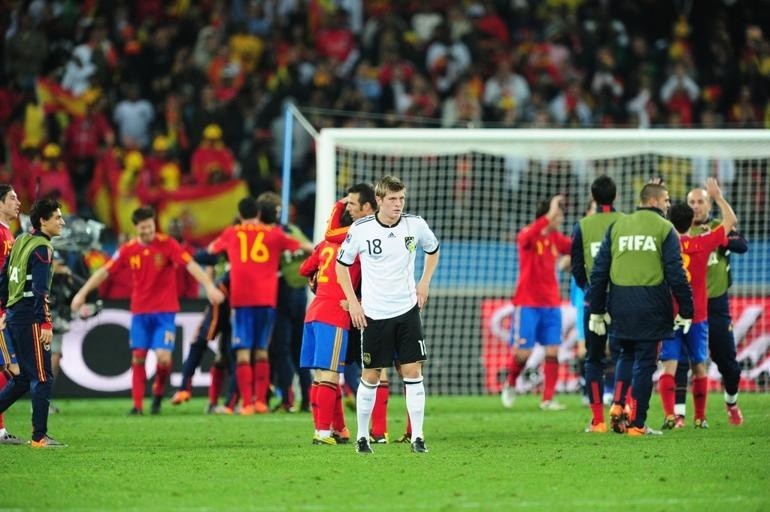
[[585, 401, 744, 436], [312, 427, 429, 453], [501, 380, 517, 408], [29, 435, 68, 447], [540, 400, 567, 412], [0, 434, 32, 445], [127, 392, 309, 416]]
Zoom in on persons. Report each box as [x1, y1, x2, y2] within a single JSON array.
[[300, 185, 411, 444], [570, 174, 748, 434], [335, 176, 440, 454], [0, 0, 769, 237], [69, 205, 224, 416], [502, 196, 572, 412], [171, 197, 315, 415], [1, 184, 67, 449]]
